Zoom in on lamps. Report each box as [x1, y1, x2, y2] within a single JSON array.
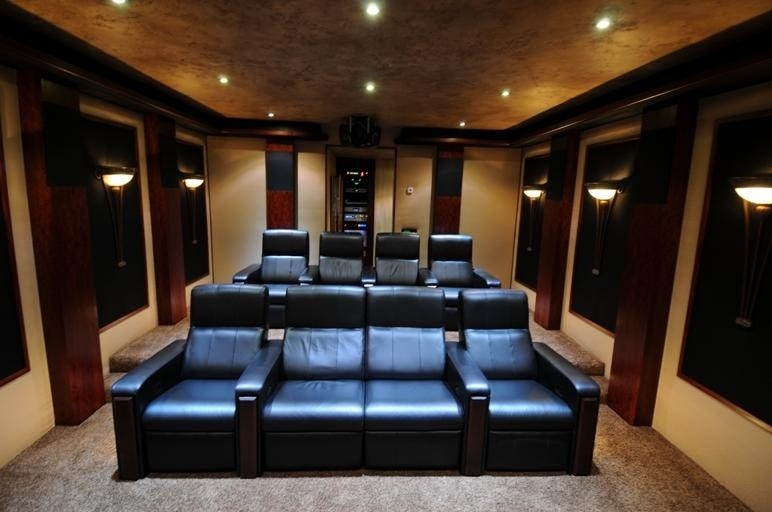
[[181, 173, 205, 245], [520, 181, 545, 252], [581, 179, 626, 277], [96, 163, 134, 270], [728, 177, 772, 336]]
